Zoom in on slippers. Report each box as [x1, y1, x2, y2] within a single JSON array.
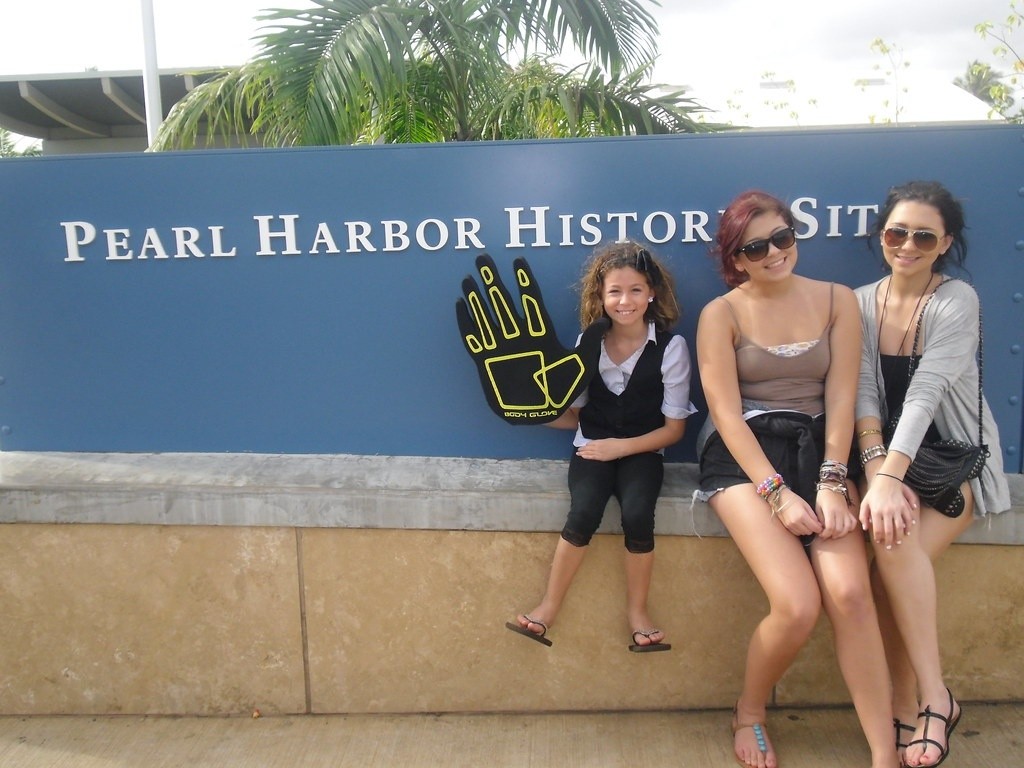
[[628, 629, 671, 653], [504, 614, 554, 647]]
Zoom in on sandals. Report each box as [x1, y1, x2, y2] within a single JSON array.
[[892, 687, 962, 768], [730, 700, 778, 768]]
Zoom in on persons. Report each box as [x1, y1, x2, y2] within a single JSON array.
[[700, 189, 898, 768], [506, 243, 699, 653]]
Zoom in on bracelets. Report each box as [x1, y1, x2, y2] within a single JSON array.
[[815, 461, 852, 507], [858, 445, 887, 469], [876, 473, 904, 484], [757, 474, 789, 515], [856, 430, 884, 439], [853, 182, 1012, 766]]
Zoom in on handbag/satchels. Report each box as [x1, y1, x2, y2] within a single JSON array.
[[882, 407, 992, 519]]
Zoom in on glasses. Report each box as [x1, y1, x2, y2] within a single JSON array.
[[733, 226, 796, 262], [882, 226, 947, 254]]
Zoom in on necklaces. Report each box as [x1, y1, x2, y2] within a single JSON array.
[[876, 271, 933, 414]]
